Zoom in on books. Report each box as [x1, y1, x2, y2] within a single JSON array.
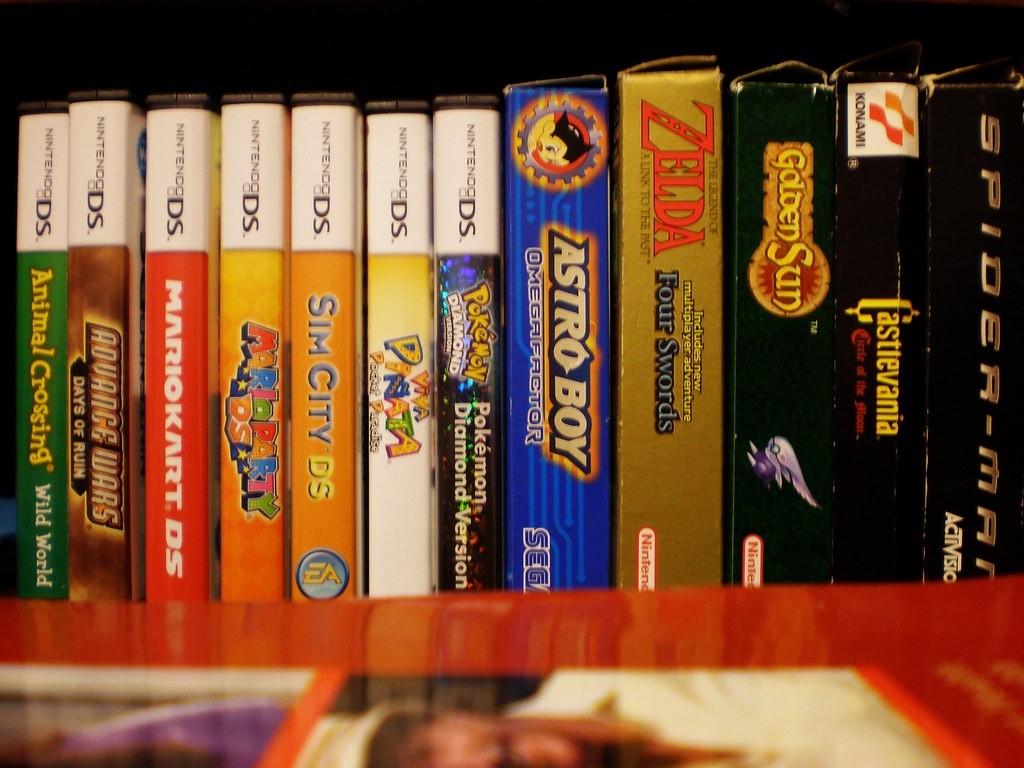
[[0, 57, 1024, 602]]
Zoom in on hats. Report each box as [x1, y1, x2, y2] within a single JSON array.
[[297, 697, 419, 768]]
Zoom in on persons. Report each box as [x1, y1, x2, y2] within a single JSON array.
[[298, 667, 943, 767]]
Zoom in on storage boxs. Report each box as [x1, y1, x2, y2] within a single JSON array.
[[15, 50, 1024, 601]]
